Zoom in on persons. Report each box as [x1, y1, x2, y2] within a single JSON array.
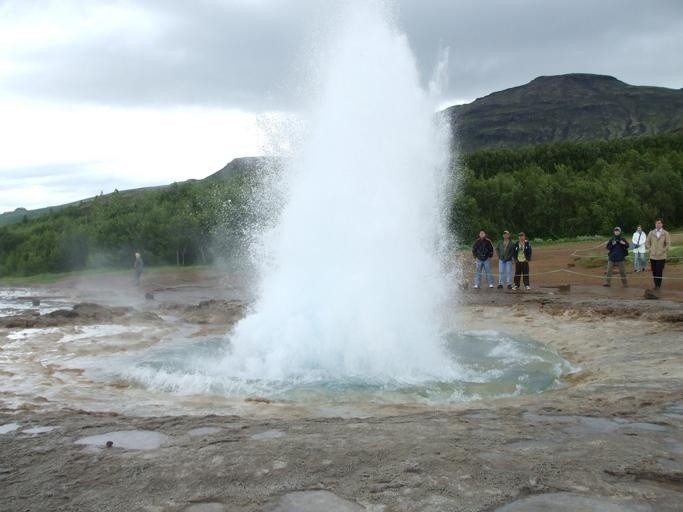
[[131, 252, 144, 287], [511, 232, 532, 291], [632, 224, 649, 273], [472, 230, 494, 289], [645, 218, 670, 290], [494, 230, 515, 289], [602, 227, 630, 288]]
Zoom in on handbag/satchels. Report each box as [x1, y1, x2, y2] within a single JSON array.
[[632, 244, 638, 248]]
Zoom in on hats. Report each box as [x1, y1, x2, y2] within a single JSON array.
[[615, 227, 621, 232], [504, 231, 509, 234]]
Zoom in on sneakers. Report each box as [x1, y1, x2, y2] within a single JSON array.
[[603, 283, 610, 287], [474, 284, 530, 291]]
